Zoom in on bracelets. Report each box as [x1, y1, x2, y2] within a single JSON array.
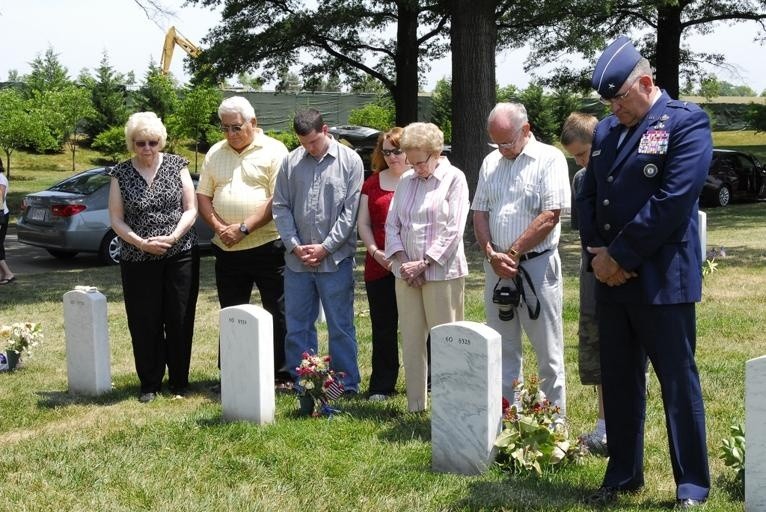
[[139, 239, 146, 249], [373, 248, 379, 258], [508, 247, 521, 258], [488, 251, 496, 263], [170, 233, 177, 242]]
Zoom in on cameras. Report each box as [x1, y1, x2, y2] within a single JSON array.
[[493, 287, 519, 321]]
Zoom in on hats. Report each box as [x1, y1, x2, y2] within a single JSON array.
[[592, 36, 642, 99]]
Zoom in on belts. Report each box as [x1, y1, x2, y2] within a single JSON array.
[[520, 249, 550, 261]]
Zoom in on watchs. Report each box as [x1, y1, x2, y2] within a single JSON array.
[[422, 257, 431, 267], [240, 222, 249, 235]]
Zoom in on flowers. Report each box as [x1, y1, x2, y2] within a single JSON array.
[[0, 317, 45, 372], [493, 372, 588, 480], [295, 349, 346, 404]]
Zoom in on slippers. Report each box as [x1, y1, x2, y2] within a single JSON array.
[[0, 275, 15, 285]]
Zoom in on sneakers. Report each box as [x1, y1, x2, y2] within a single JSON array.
[[588, 435, 609, 456], [362, 391, 390, 401], [343, 391, 357, 398]]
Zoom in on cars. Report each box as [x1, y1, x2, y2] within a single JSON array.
[[328, 122, 383, 177], [439, 144, 451, 158], [14, 165, 217, 265], [697, 149, 763, 209]]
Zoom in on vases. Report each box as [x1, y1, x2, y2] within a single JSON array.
[[5, 349, 28, 371], [297, 396, 314, 417]]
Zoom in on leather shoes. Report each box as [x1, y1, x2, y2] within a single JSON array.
[[166, 383, 190, 396], [139, 390, 156, 403], [676, 499, 706, 509], [584, 485, 642, 503], [275, 371, 293, 392]]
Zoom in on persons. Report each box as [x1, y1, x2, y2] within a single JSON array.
[[561, 109, 610, 447], [356, 126, 431, 401], [271, 107, 365, 400], [383, 121, 471, 415], [194, 94, 290, 393], [0, 158, 15, 285], [470, 99, 572, 466], [108, 111, 199, 403], [577, 34, 713, 508]]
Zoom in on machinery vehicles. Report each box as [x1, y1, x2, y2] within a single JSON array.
[[155, 24, 254, 93]]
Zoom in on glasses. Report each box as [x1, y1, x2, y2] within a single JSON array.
[[220, 121, 245, 133], [600, 77, 642, 105], [404, 152, 433, 168], [381, 148, 401, 156], [488, 130, 524, 148], [136, 141, 158, 146]]
